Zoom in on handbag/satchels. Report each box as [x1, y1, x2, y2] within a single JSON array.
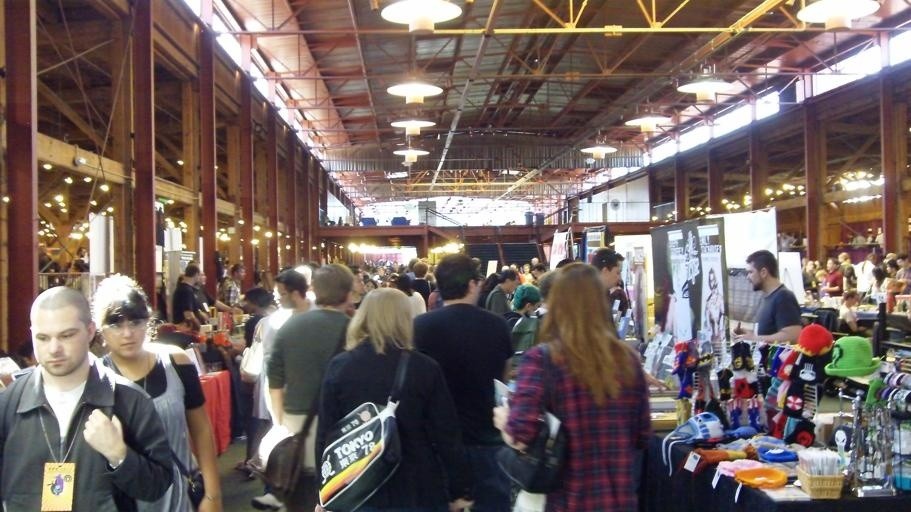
[[318, 402, 403, 511], [188, 472, 205, 508], [493, 419, 571, 492], [239, 340, 264, 385], [264, 432, 304, 503]]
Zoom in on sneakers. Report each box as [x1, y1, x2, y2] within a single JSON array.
[[252, 493, 284, 511]]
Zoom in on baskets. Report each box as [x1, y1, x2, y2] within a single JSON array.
[[796, 466, 846, 499]]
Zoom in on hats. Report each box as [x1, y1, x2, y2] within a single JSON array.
[[792, 324, 881, 377], [662, 414, 798, 489]]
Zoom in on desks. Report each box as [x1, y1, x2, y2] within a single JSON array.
[[799, 294, 882, 322], [185, 370, 232, 462], [646, 424, 911, 512], [174, 327, 254, 439]]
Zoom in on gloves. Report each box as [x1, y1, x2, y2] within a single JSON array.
[[669, 341, 803, 419]]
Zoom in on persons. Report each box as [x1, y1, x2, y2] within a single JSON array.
[[173, 261, 246, 329], [240, 253, 653, 510], [1, 273, 215, 512], [729, 250, 804, 345], [802, 253, 911, 333], [703, 269, 726, 365]]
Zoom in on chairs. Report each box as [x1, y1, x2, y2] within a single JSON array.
[[811, 309, 852, 337]]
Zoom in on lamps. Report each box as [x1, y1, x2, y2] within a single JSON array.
[[580, 129, 617, 160], [623, 100, 673, 132], [796, 1, 882, 32], [677, 62, 735, 101], [377, 0, 464, 164]]
